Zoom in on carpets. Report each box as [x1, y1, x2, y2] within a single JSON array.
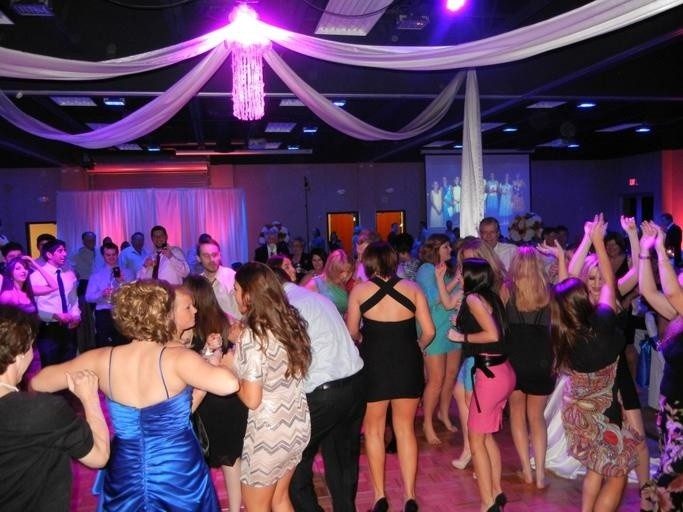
[[151, 252, 162, 280], [56, 269, 67, 313]]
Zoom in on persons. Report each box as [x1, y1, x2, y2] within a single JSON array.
[[228, 260, 311, 509], [1, 226, 246, 368], [29, 280, 240, 508], [1, 317, 111, 510], [256, 212, 683, 510], [428, 170, 527, 228]]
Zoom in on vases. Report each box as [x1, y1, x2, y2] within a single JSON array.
[[257, 220, 291, 247], [508, 211, 543, 245]]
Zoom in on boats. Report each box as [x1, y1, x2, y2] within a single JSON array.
[[641, 196, 653, 223], [623, 197, 636, 225]]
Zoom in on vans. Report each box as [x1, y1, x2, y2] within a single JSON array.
[[487, 490, 506, 511]]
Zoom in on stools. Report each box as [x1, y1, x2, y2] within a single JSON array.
[[41, 320, 59, 327], [314, 369, 361, 392]]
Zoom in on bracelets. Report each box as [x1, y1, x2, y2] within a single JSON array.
[[638, 253, 650, 259], [464, 333, 469, 342]]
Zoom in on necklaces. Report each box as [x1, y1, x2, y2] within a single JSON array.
[[2, 382, 21, 392]]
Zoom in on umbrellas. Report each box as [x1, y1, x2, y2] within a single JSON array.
[[367, 497, 389, 512], [452, 454, 473, 470], [405, 499, 417, 512]]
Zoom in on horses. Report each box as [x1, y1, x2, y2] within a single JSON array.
[[113, 267, 120, 278], [162, 241, 167, 249]]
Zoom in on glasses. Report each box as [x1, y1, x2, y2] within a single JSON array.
[[226, 2, 267, 121]]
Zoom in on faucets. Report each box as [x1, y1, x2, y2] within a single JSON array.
[[436, 411, 458, 433], [422, 424, 443, 448]]
